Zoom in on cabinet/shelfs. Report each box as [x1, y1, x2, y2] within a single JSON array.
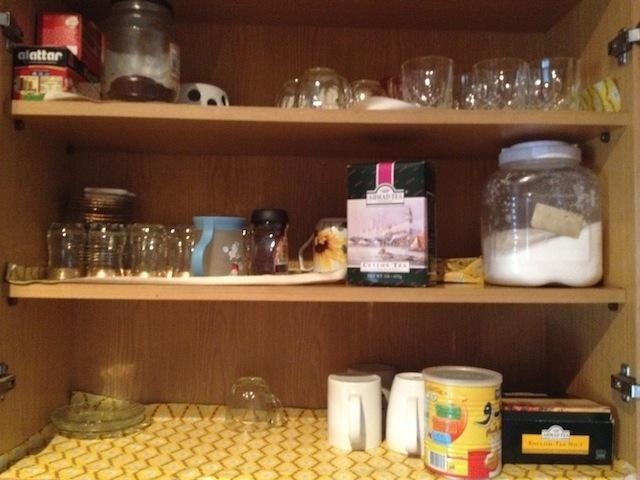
[[1, 0, 640, 480]]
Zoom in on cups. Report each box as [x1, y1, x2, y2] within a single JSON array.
[[225, 376, 288, 432], [457, 72, 489, 109], [47, 219, 200, 280], [326, 374, 382, 450], [385, 371, 425, 455], [346, 80, 386, 112], [465, 57, 529, 112], [294, 67, 348, 109], [278, 77, 300, 109], [298, 217, 346, 272], [525, 56, 583, 111], [400, 57, 454, 111], [204, 230, 248, 276]]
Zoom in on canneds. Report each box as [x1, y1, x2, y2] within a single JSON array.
[[422, 365, 503, 480]]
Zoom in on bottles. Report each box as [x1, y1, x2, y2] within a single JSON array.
[[252, 207, 290, 271], [481, 141, 603, 288], [102, 1, 182, 102], [187, 214, 246, 278]]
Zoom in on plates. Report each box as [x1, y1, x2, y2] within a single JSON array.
[[50, 398, 145, 434]]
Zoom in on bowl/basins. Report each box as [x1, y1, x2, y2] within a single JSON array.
[[81, 186, 138, 217]]
